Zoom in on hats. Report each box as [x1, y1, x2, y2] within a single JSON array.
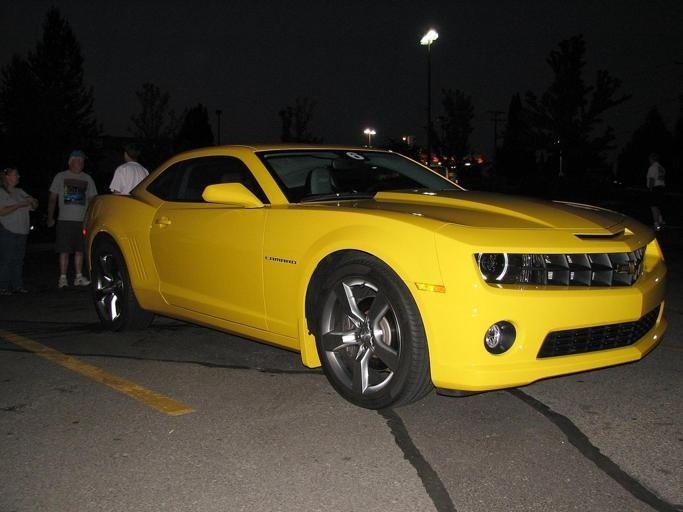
[[70, 150, 89, 160]]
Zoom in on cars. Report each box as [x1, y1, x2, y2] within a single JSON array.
[[81, 143, 669, 410]]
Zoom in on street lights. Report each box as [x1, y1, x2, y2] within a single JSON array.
[[364, 127, 376, 146], [420, 28, 439, 166]]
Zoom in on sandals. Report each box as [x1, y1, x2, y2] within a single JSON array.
[[0, 288, 29, 296]]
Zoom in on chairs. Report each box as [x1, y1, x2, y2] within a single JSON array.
[[305, 167, 336, 197]]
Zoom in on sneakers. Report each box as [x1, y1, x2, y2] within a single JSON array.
[[58, 275, 92, 288]]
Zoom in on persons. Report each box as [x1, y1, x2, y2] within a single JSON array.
[[646, 153, 667, 231], [0, 166, 39, 297], [45, 150, 98, 288], [109, 143, 149, 195]]
